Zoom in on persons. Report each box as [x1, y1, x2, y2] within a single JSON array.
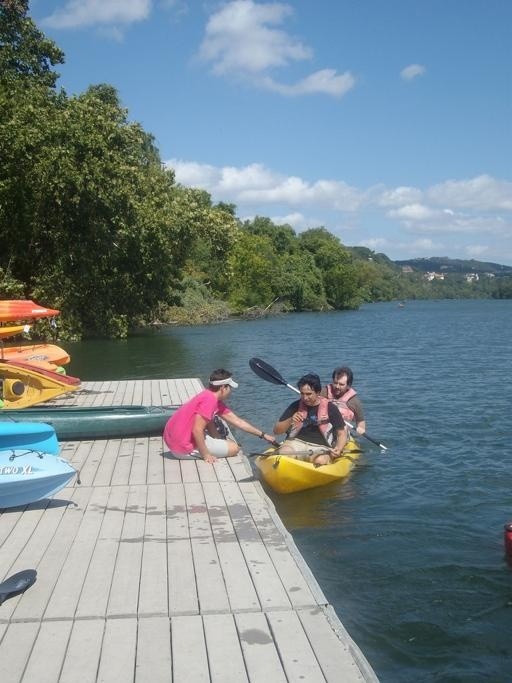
[[273, 374, 347, 466], [163, 368, 276, 464], [319, 367, 365, 436]]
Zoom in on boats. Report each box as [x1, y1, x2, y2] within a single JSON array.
[[0, 450, 78, 508], [0, 360, 81, 410], [0, 299, 60, 321], [0, 345, 71, 367], [255, 411, 361, 495], [1, 403, 186, 441], [0, 323, 35, 339]]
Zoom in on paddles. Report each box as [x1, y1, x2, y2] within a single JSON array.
[[249, 358, 388, 446], [247, 449, 364, 458]]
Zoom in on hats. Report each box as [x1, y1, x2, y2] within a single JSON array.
[[208, 376, 239, 389]]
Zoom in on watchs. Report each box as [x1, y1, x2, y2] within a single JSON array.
[[259, 432, 265, 439]]
